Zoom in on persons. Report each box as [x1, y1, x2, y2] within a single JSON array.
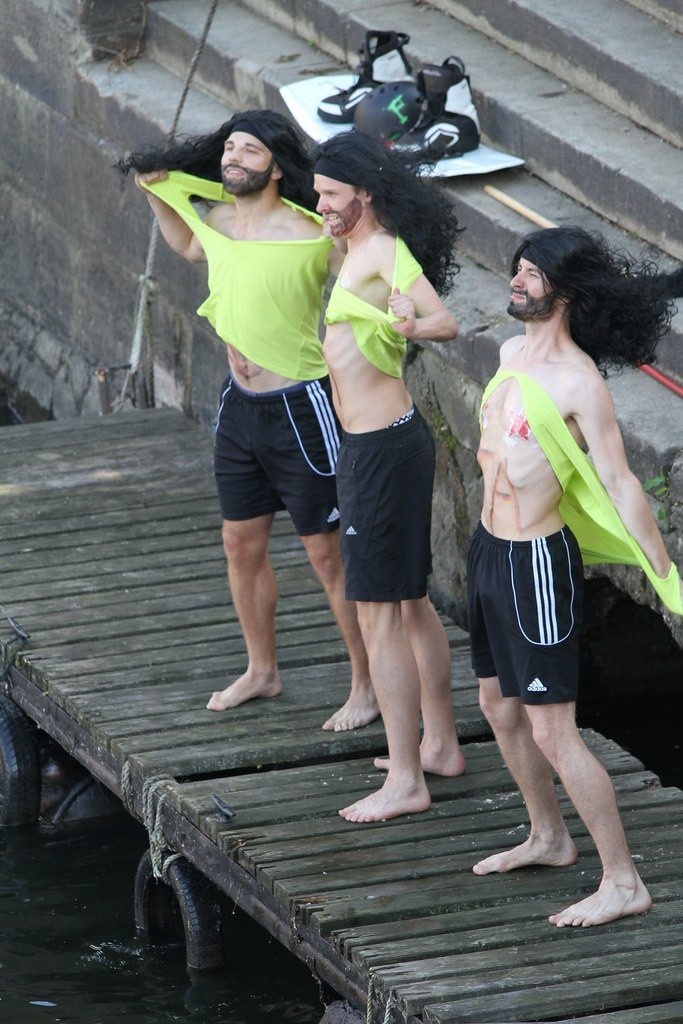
[[466, 226, 682, 928], [313, 131, 464, 824], [132, 110, 379, 732]]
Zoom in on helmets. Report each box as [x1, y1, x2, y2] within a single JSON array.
[[353, 80, 425, 145]]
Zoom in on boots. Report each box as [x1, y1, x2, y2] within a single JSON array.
[[384, 56, 481, 164], [317, 30, 410, 120]]
